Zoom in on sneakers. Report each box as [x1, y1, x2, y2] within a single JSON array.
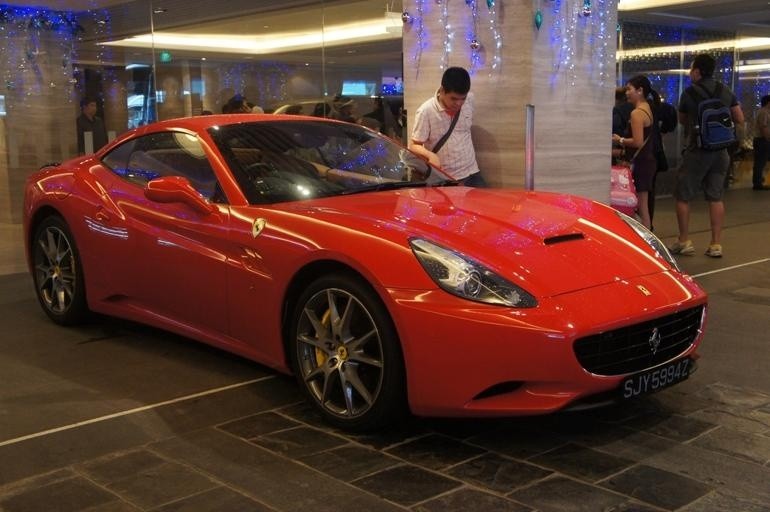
[[751, 183, 770, 191], [666, 235, 697, 258], [703, 243, 724, 258]]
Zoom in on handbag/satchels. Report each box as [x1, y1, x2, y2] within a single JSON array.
[[616, 157, 635, 173]]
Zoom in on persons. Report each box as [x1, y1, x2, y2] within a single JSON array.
[[77, 96, 106, 156], [198, 92, 403, 168], [410, 66, 486, 188], [613, 51, 770, 257]]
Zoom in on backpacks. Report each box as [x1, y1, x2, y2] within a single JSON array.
[[684, 81, 737, 152]]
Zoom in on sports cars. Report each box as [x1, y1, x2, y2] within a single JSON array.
[[21, 109, 710, 429]]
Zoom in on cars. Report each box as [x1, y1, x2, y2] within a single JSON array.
[[272, 93, 408, 186]]
